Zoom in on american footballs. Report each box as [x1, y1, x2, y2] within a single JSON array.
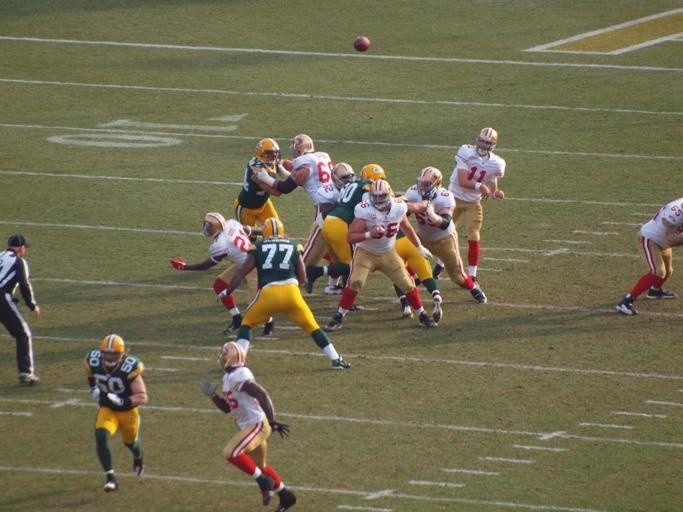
[[354, 37, 370, 51]]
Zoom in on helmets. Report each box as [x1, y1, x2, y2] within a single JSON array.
[[101, 333, 125, 367], [256, 137, 279, 163], [218, 341, 243, 370], [417, 166, 443, 196], [263, 217, 284, 239], [332, 162, 392, 210], [290, 133, 314, 157], [203, 212, 225, 238], [475, 127, 498, 156]]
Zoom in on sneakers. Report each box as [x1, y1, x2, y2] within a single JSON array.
[[274, 492, 296, 512], [470, 281, 487, 303], [325, 311, 343, 332], [133, 452, 144, 476], [223, 313, 242, 335], [324, 283, 342, 294], [307, 264, 316, 293], [646, 288, 676, 300], [402, 294, 443, 326], [616, 299, 638, 316], [259, 475, 275, 506], [19, 373, 41, 382], [105, 481, 117, 492], [263, 316, 274, 335], [332, 356, 351, 370]]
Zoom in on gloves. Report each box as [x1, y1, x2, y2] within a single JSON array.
[[425, 200, 438, 223], [252, 166, 275, 188], [107, 392, 124, 406], [91, 386, 101, 399], [270, 418, 289, 439], [169, 256, 185, 270], [216, 289, 227, 302], [197, 377, 220, 398], [416, 244, 432, 260]]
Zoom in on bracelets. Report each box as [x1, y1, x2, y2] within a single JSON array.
[[474, 182, 480, 190], [270, 421, 278, 426]]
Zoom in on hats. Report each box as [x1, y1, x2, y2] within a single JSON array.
[[8, 234, 32, 246]]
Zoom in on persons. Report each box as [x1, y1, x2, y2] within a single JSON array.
[[233, 139, 285, 239], [614, 197, 683, 316], [255, 134, 340, 220], [81, 334, 147, 491], [216, 218, 351, 370], [168, 212, 286, 337], [0, 235, 40, 383], [405, 167, 487, 303], [442, 128, 506, 288], [299, 161, 442, 332], [199, 342, 296, 511]]
